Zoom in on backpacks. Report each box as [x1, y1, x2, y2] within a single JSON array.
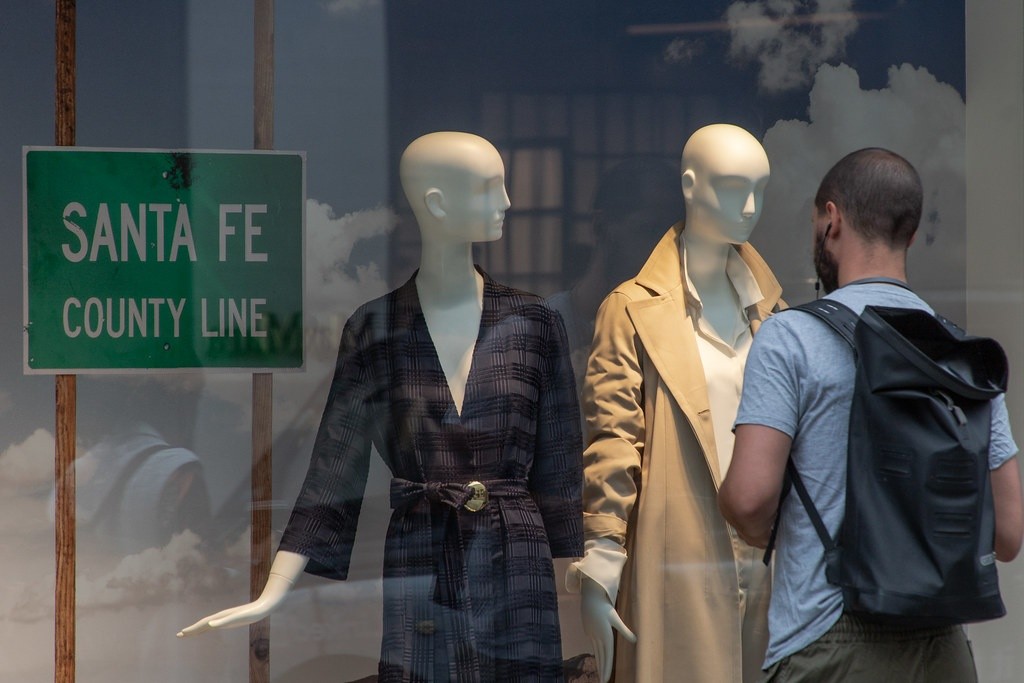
[[778, 299, 1008, 624]]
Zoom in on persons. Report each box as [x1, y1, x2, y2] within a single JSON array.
[[177, 131, 584, 683], [717, 148, 1024, 683], [48, 370, 225, 606], [564, 125, 790, 683]]
